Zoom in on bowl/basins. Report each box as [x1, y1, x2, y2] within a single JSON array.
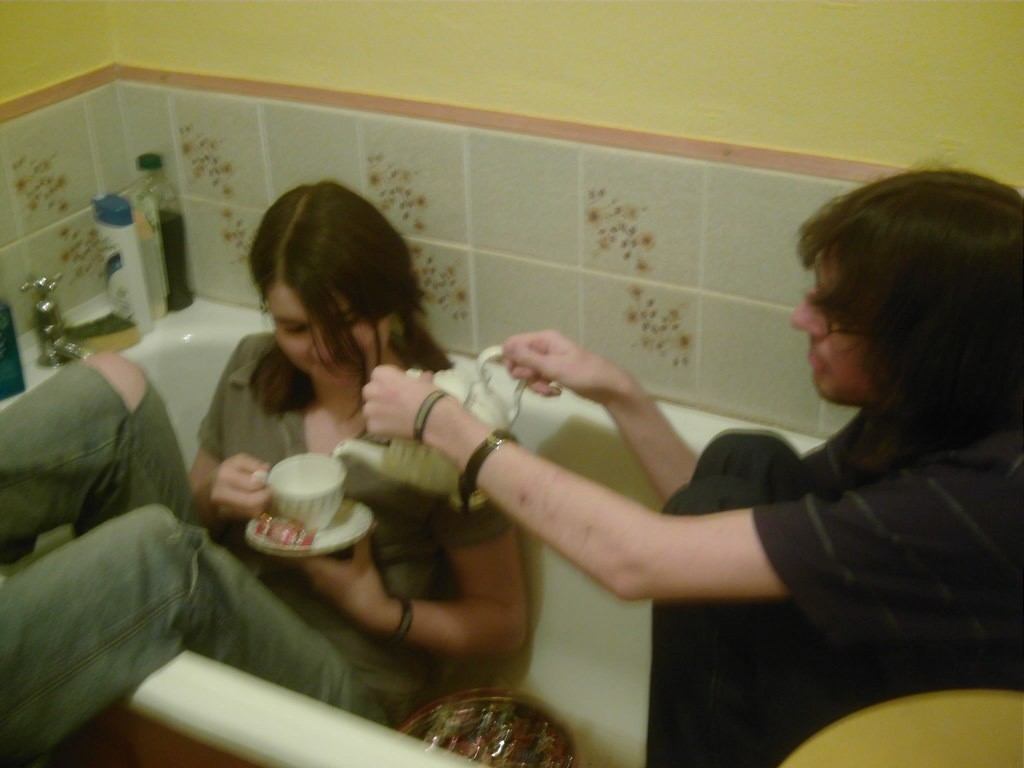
[[403, 689, 577, 768]]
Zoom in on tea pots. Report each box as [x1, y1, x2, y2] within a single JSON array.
[[333, 345, 526, 509]]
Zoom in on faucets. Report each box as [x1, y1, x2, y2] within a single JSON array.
[[20, 272, 92, 366]]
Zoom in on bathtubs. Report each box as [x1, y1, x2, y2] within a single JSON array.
[[0, 286, 832, 768]]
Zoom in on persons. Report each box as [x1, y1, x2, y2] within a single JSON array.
[[0, 181, 527, 768], [361, 169, 1024, 768]]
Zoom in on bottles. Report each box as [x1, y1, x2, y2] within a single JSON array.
[[130, 155, 194, 312]]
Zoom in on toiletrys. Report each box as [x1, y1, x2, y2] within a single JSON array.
[[88, 190, 158, 339], [129, 151, 195, 315]]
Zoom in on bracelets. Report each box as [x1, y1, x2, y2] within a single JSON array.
[[412, 390, 448, 445], [384, 595, 412, 648], [455, 428, 517, 519]]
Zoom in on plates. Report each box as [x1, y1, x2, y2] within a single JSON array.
[[246, 501, 375, 557]]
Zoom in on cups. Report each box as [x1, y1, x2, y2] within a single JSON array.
[[252, 454, 349, 532]]
